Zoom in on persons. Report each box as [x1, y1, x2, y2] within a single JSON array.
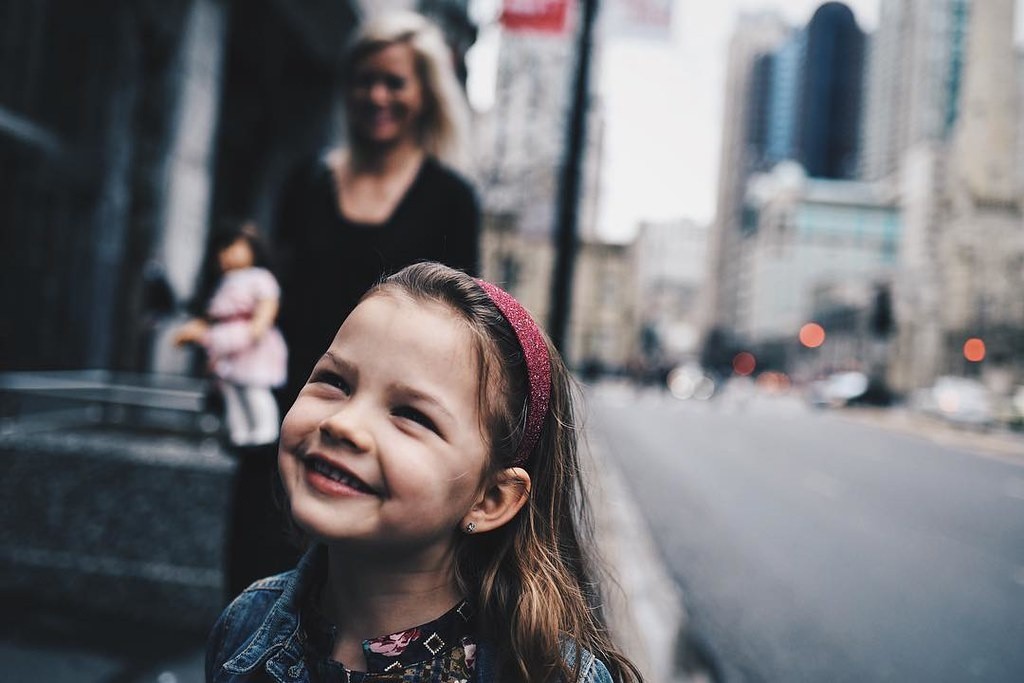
[[170, 223, 290, 446], [221, 9, 484, 598], [204, 263, 646, 682]]
